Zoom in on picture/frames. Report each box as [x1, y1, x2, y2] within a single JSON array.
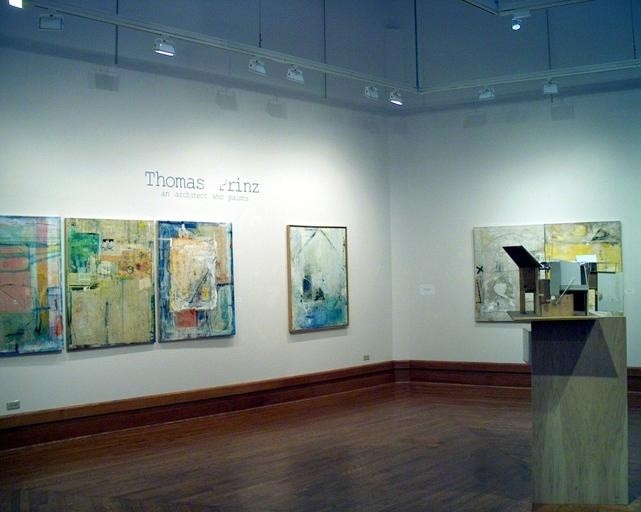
[[285, 224, 350, 332]]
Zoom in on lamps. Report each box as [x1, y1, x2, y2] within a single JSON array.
[[510, 10, 534, 32], [34, 6, 408, 109]]
